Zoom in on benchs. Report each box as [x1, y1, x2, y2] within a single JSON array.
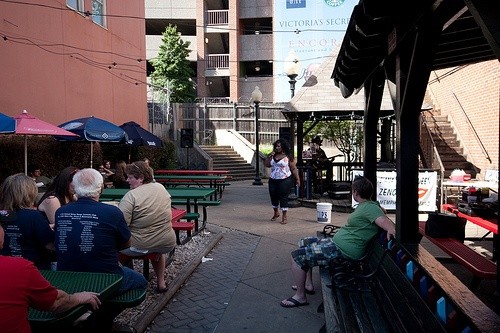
[[316, 229, 500, 333], [419, 221, 498, 280], [96, 175, 232, 306]]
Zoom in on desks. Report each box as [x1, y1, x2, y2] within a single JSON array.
[[154, 176, 226, 200], [155, 170, 228, 192], [443, 179, 498, 215], [26, 271, 124, 333], [100, 189, 216, 240], [443, 204, 498, 232], [172, 209, 187, 223]]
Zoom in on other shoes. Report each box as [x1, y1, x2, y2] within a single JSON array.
[[87, 314, 120, 330]]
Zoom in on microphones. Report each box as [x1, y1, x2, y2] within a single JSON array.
[[268, 149, 278, 158]]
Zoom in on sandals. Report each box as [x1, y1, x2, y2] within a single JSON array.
[[271, 214, 280, 221], [281, 217, 288, 224]]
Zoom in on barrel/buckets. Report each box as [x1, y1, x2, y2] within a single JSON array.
[[316, 203, 332, 224]]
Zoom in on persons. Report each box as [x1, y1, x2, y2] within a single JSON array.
[[280, 176, 395, 307], [265, 140, 301, 224], [0, 157, 176, 333]]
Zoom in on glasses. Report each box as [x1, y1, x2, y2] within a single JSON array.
[[70, 169, 80, 175]]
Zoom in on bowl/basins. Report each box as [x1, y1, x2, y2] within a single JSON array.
[[450, 175, 464, 183]]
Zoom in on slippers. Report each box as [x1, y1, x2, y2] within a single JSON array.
[[157, 281, 169, 291], [280, 297, 308, 308], [291, 284, 315, 294]]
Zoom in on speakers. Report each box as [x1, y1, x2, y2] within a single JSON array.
[[279, 127, 294, 159], [180, 128, 193, 148]]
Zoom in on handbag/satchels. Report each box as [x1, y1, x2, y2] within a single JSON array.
[[329, 255, 371, 286], [425, 210, 467, 241]]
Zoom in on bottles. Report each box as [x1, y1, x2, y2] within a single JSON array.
[[468, 187, 477, 204]]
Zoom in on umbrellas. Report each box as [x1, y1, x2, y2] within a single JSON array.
[[0, 109, 163, 176]]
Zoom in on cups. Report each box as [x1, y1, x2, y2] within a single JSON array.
[[464, 174, 471, 183], [476, 174, 484, 183]]
